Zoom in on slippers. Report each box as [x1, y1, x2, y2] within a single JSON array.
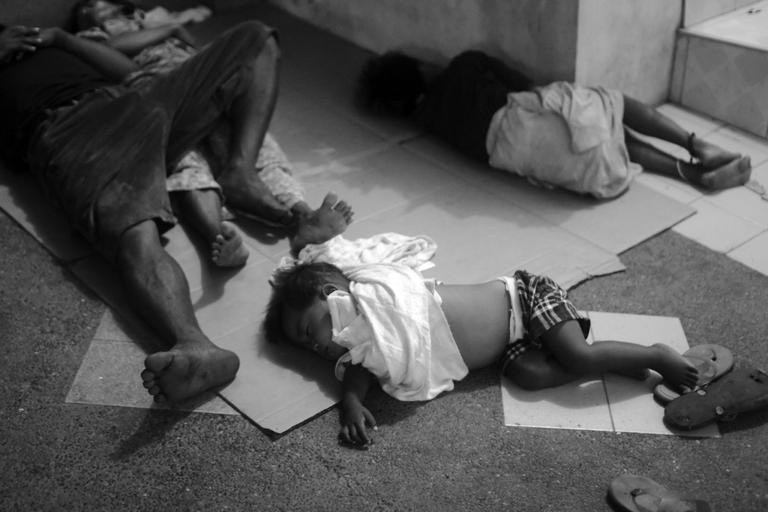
[[610, 474, 712, 512], [665, 367, 768, 429], [654, 344, 735, 401]]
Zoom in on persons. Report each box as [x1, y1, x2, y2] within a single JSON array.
[[352, 50, 751, 200], [70, 0, 355, 269], [262, 262, 700, 448], [0, 18, 295, 404]]
